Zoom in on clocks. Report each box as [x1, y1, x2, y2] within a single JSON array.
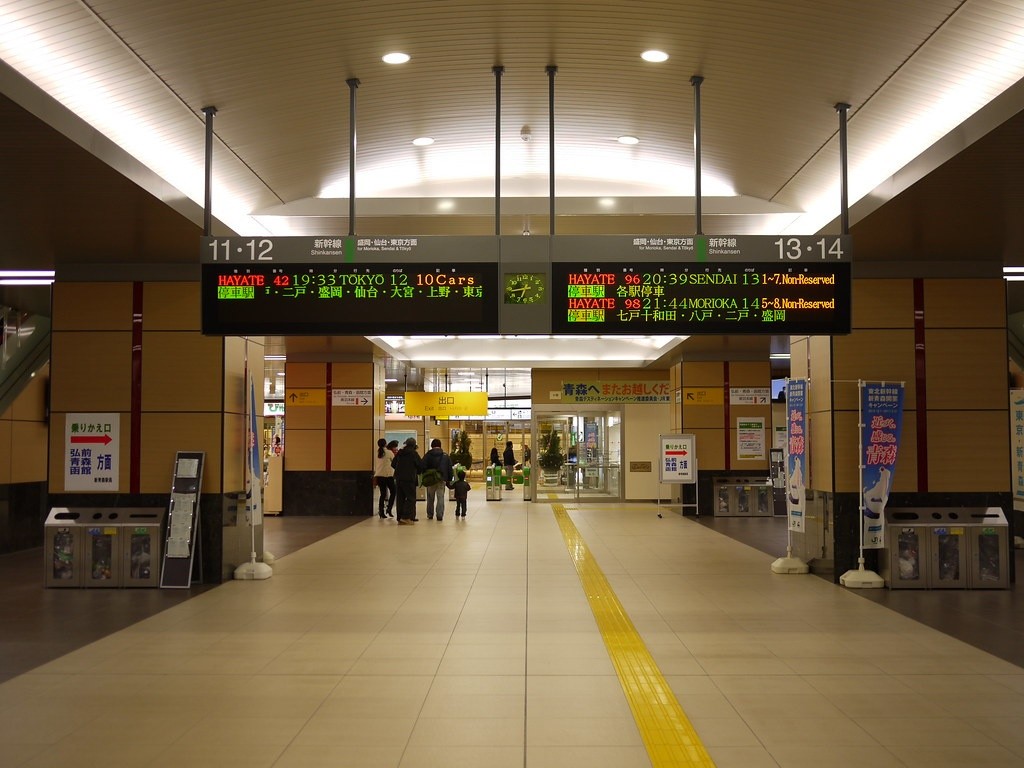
[[505, 273, 543, 304]]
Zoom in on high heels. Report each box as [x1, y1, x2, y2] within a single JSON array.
[[386, 509, 394, 517], [379, 512, 387, 519]]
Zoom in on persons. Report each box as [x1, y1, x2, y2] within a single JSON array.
[[375, 438, 453, 524], [525, 445, 531, 467], [490, 447, 503, 468], [447, 471, 470, 516], [503, 441, 517, 490], [564, 446, 577, 463]]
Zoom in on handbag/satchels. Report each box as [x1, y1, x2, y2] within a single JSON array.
[[419, 468, 442, 488], [372, 477, 377, 488]]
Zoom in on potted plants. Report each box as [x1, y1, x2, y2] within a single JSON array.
[[538, 430, 565, 484]]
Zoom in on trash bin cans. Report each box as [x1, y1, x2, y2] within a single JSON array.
[[878, 507, 1009, 591], [43, 507, 167, 589], [713, 476, 774, 517]]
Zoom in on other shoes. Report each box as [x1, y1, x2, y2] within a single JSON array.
[[456, 513, 460, 516], [411, 516, 418, 521], [505, 487, 514, 490], [427, 515, 433, 519], [437, 517, 442, 520], [461, 514, 466, 516]]
